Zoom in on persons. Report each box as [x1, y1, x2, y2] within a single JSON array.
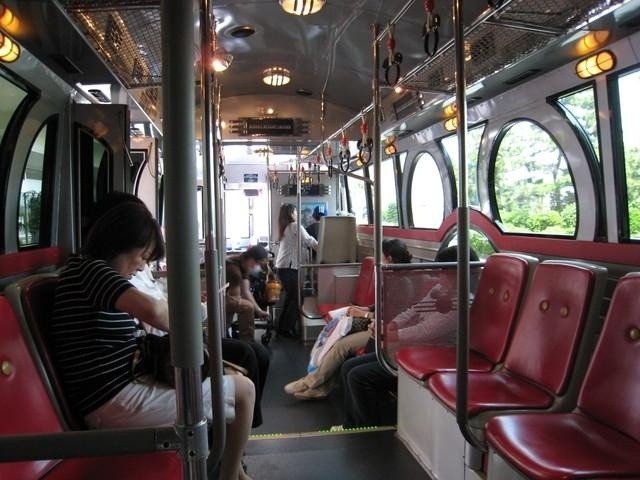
[[305, 204, 328, 273], [272, 203, 319, 334], [284, 237, 413, 402], [106, 193, 281, 431], [51, 193, 256, 479], [330, 244, 479, 431]]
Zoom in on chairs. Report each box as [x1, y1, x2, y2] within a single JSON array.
[[393, 250, 540, 381], [426, 257, 611, 420], [1, 267, 184, 480], [480, 269, 640, 479], [312, 258, 435, 363]]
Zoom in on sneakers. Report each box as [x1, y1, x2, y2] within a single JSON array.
[[283, 374, 328, 400]]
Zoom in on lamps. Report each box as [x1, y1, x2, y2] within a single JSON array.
[[576, 50, 617, 78], [1, 33, 21, 62], [213, 0, 325, 118]]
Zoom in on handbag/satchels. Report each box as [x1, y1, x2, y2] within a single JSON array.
[[136, 328, 210, 390]]
